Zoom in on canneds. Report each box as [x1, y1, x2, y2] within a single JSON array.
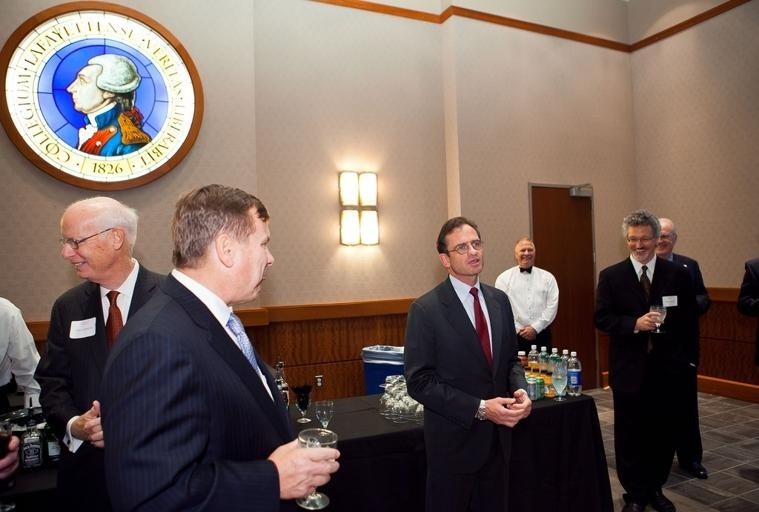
[[524, 373, 545, 402]]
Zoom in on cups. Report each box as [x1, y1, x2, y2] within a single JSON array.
[[291, 384, 311, 424]]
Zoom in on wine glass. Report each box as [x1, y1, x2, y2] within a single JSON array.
[[314, 400, 334, 437], [552, 361, 567, 402], [379, 374, 425, 425], [295, 428, 340, 510]]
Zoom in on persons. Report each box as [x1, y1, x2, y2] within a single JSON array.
[[739, 257, 759, 329], [0, 295, 44, 480], [68, 55, 151, 156], [652, 217, 712, 480], [100, 184, 342, 512], [34, 197, 172, 512], [403, 216, 532, 511], [494, 238, 559, 355], [594, 209, 700, 512]]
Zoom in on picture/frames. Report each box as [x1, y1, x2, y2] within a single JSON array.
[[1, 2, 205, 192]]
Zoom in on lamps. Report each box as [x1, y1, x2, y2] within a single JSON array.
[[340, 171, 379, 247]]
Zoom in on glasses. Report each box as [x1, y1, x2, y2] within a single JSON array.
[[627, 236, 655, 242], [448, 241, 481, 255], [59, 228, 114, 250]]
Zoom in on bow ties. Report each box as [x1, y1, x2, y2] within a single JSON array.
[[520, 267, 532, 274]]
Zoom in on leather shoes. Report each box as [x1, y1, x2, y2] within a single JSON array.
[[621, 501, 646, 511], [693, 466, 708, 479], [648, 493, 676, 512]]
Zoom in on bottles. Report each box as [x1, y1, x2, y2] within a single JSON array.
[[567, 352, 583, 397], [524, 366, 547, 378], [42, 420, 63, 469], [545, 373, 556, 397], [274, 362, 289, 419], [651, 305, 667, 335], [0, 419, 11, 460], [528, 345, 539, 366], [559, 349, 571, 362], [550, 348, 562, 367], [19, 421, 44, 469], [540, 346, 549, 365], [316, 375, 325, 424], [517, 350, 527, 368]]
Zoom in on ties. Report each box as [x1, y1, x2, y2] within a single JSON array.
[[227, 313, 259, 375], [639, 266, 652, 353], [469, 287, 494, 369], [105, 291, 123, 351]]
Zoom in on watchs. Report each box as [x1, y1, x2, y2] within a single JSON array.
[[477, 399, 486, 421]]
